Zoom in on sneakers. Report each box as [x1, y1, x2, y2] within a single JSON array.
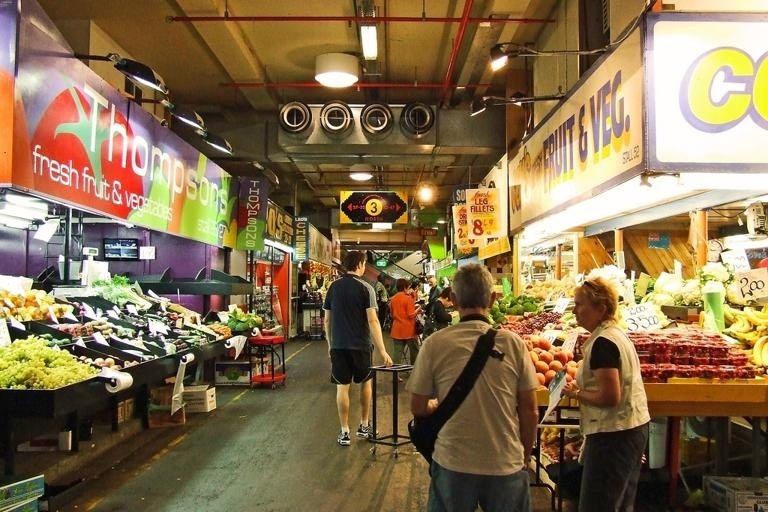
[[337, 431, 351, 446], [356, 422, 378, 438]]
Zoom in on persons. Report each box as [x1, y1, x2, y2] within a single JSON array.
[[323, 251, 394, 446], [562, 277, 651, 510], [374, 275, 454, 381], [404, 264, 539, 511]]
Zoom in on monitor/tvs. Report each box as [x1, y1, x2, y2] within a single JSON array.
[[103, 237, 139, 262]]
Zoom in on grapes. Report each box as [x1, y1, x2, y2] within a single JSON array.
[[0, 336, 102, 390]]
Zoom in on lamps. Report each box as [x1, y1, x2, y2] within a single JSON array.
[[510, 91, 534, 139], [74, 52, 169, 96], [490, 0, 659, 72], [160, 116, 234, 153], [120, 87, 207, 133], [358, 0, 378, 62], [315, 53, 359, 88], [349, 163, 374, 181], [470, 85, 565, 115]]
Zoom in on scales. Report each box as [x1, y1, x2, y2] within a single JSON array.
[[51, 247, 98, 297]]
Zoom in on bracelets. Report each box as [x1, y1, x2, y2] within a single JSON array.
[[574, 389, 581, 399]]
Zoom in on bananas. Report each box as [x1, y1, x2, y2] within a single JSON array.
[[722, 303, 768, 367]]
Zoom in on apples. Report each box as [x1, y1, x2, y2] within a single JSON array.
[[524, 338, 583, 386]]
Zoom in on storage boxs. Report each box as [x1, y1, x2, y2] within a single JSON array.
[[214, 358, 268, 386], [702, 474, 768, 512], [148, 384, 217, 429]]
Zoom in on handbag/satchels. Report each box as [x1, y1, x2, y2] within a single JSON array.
[[425, 318, 448, 335], [416, 320, 423, 335], [407, 414, 435, 460]]
